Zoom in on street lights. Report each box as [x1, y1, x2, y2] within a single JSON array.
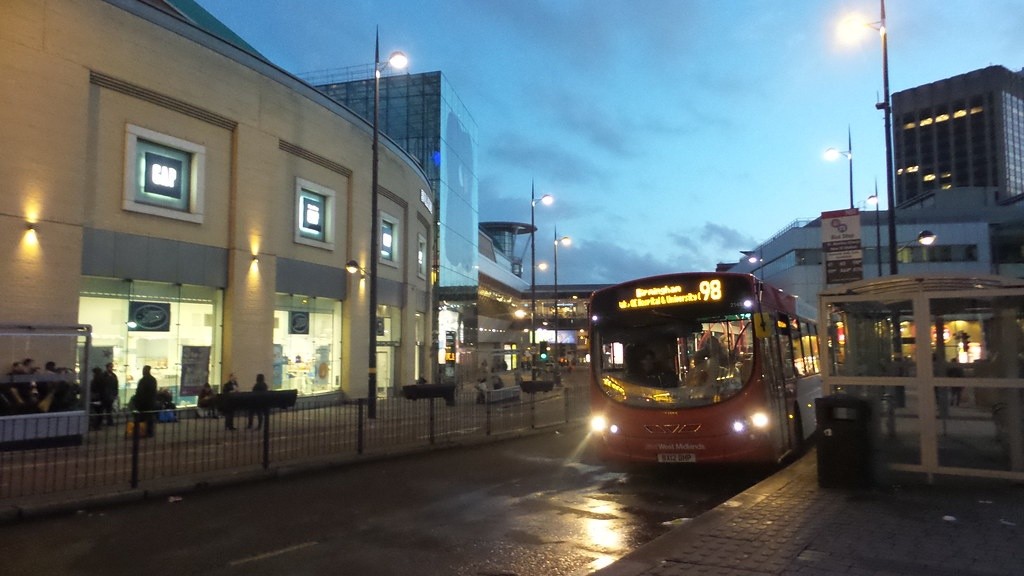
[[346, 51, 407, 417], [515, 194, 554, 381], [835, 8, 937, 409], [866, 194, 882, 277], [543, 236, 571, 385], [825, 146, 854, 209], [748, 257, 764, 281]]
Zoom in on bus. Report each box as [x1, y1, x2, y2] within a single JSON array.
[[586, 272, 823, 464]]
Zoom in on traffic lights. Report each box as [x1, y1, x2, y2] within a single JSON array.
[[560, 343, 566, 357], [539, 341, 547, 359]]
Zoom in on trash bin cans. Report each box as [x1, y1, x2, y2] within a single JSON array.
[[815, 395, 881, 489], [554, 371, 562, 385]]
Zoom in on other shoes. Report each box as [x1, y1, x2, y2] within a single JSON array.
[[225, 424, 236, 431], [245, 426, 253, 429], [255, 426, 261, 431], [212, 415, 219, 419]]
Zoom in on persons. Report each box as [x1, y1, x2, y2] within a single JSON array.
[[223, 373, 240, 430], [475, 359, 576, 404], [417, 373, 426, 383], [882, 353, 965, 415], [248, 374, 267, 430], [688, 330, 799, 404], [0, 359, 118, 431], [197, 382, 219, 419], [135, 365, 157, 438]]
